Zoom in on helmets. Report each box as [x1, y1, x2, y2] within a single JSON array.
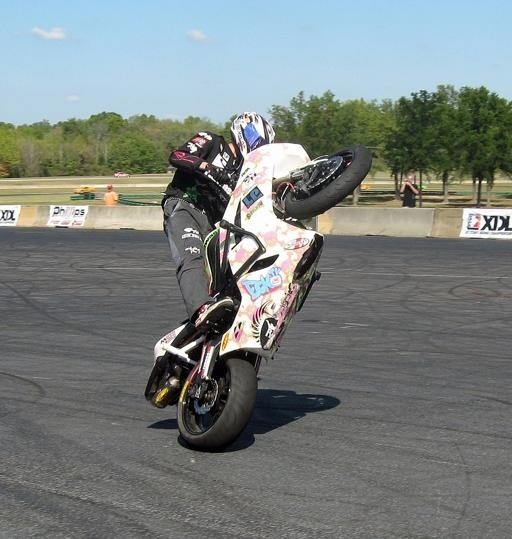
[[230, 112, 275, 158]]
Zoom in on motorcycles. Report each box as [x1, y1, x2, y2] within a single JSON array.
[[141, 136, 376, 452]]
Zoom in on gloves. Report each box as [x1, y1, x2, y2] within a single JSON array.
[[204, 164, 231, 185]]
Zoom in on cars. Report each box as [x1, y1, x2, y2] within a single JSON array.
[[73, 184, 98, 194], [360, 184, 371, 190], [417, 185, 427, 189], [114, 171, 131, 176], [69, 192, 96, 200]]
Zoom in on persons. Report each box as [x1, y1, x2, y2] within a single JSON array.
[[160, 111, 272, 336], [397, 175, 422, 208], [101, 186, 121, 206]]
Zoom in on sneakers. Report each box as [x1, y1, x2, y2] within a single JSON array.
[[195, 299, 233, 328]]
